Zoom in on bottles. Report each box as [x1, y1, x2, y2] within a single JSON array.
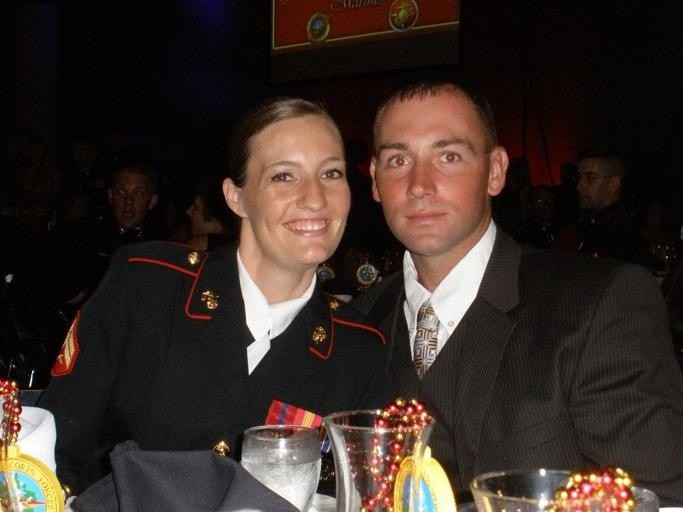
[[322, 410, 435, 512]]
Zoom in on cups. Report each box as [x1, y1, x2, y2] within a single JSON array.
[[629, 486, 658, 512], [466, 468, 607, 512], [237, 423, 322, 512]]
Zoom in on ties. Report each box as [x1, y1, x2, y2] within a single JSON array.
[[414, 299, 439, 381]]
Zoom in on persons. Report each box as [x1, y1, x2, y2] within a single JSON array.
[[346, 72, 682, 510], [0, 120, 239, 390], [492, 142, 683, 283], [33, 92, 392, 500]]
